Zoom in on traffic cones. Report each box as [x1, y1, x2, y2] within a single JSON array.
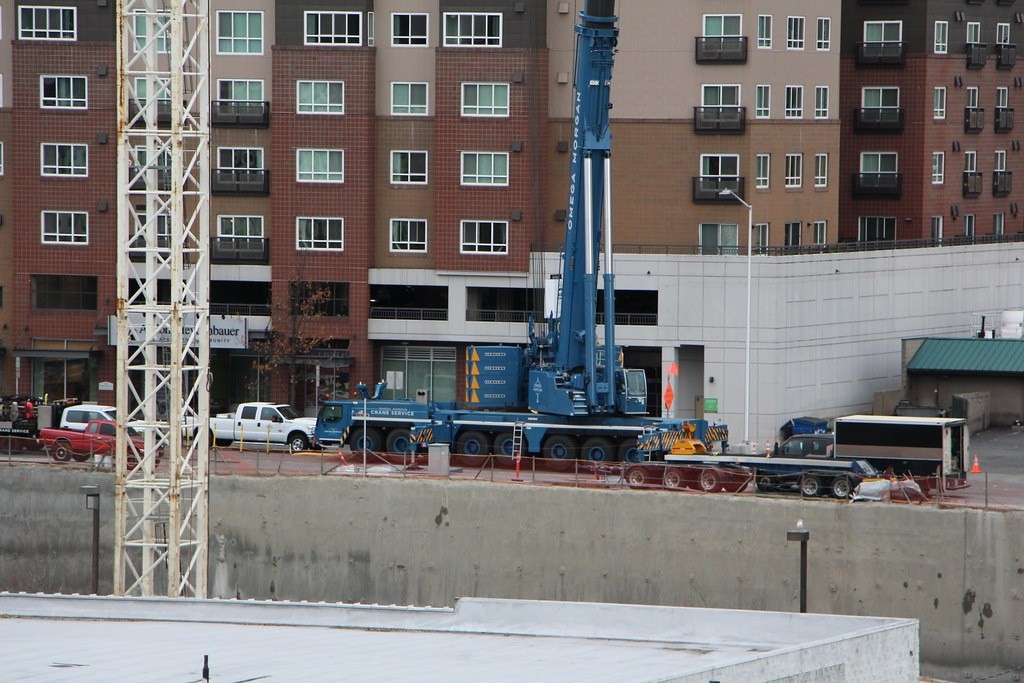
[[972, 454, 980, 473]]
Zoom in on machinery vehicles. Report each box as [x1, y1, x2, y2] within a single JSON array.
[[312, 1, 728, 477]]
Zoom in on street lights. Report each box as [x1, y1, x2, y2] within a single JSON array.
[[788, 530, 812, 610], [716, 189, 753, 443], [81, 484, 102, 595]]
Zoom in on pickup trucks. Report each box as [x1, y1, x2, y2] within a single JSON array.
[[39, 419, 163, 471], [182, 401, 319, 452]]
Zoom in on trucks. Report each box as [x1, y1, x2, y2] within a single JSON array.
[[0, 393, 162, 452], [767, 413, 972, 497]]
